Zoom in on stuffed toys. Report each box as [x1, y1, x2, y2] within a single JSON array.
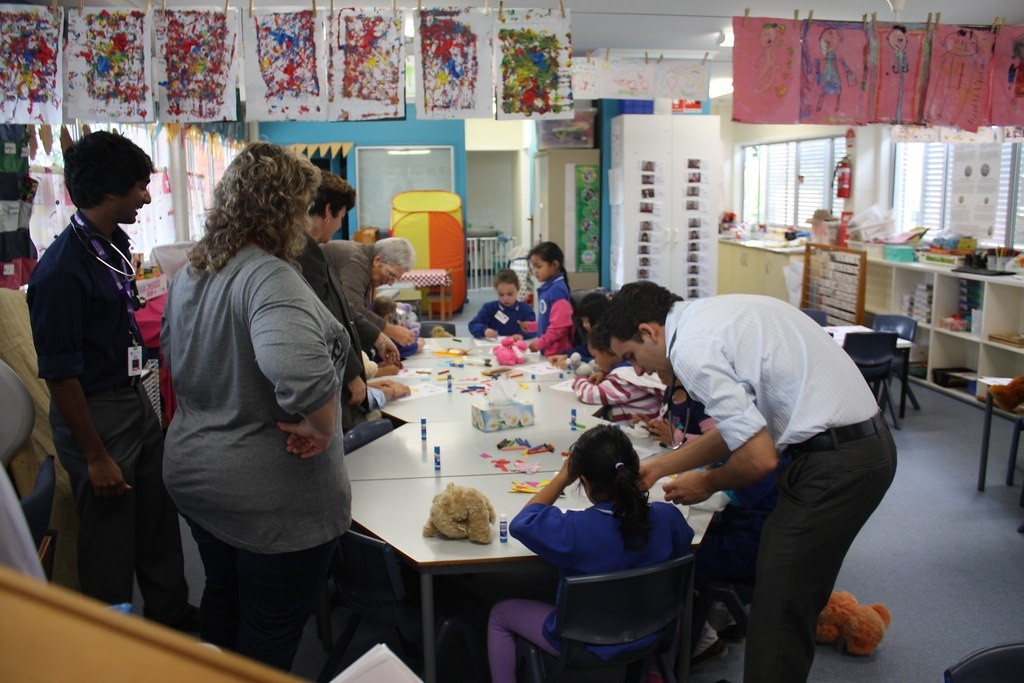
[[492, 338, 527, 366], [568, 351, 600, 375], [816, 591, 892, 656], [988, 375, 1024, 411], [423, 482, 496, 545], [394, 302, 453, 338]]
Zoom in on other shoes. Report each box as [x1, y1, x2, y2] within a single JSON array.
[[646, 670, 663, 683], [166, 606, 200, 631]]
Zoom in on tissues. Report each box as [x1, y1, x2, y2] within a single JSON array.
[[470, 380, 535, 434]]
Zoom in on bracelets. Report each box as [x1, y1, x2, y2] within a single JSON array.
[[388, 385, 396, 399], [377, 336, 390, 349]]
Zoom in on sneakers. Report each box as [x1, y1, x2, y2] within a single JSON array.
[[675, 628, 729, 670]]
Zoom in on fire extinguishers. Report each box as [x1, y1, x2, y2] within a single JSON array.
[[831, 154, 851, 198]]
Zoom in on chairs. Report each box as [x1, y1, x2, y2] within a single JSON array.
[[514, 552, 699, 683], [314, 417, 481, 683], [19, 455, 60, 582], [841, 330, 902, 433], [870, 315, 921, 419]]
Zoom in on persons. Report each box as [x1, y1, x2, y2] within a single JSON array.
[[294, 170, 426, 433], [26, 131, 201, 634], [133, 361, 139, 370], [160, 141, 350, 674], [488, 424, 696, 683], [637, 160, 701, 297], [469, 242, 780, 669], [604, 281, 898, 683]]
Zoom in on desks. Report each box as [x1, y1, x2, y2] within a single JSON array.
[[976, 376, 1024, 533], [339, 334, 722, 683], [821, 322, 913, 350], [399, 268, 452, 320]]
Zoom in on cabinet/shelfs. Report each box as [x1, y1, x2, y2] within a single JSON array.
[[863, 258, 1024, 423], [717, 242, 805, 302]]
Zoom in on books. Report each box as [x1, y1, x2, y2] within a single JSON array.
[[987, 331, 1023, 350]]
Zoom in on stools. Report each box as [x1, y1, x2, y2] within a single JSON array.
[[426, 292, 453, 321]]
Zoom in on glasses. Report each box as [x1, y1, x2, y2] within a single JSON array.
[[386, 272, 396, 282]]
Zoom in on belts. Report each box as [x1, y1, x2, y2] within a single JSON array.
[[792, 412, 885, 452], [104, 375, 140, 389]]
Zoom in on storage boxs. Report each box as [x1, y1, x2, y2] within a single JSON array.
[[931, 366, 976, 388], [535, 107, 599, 151]]
